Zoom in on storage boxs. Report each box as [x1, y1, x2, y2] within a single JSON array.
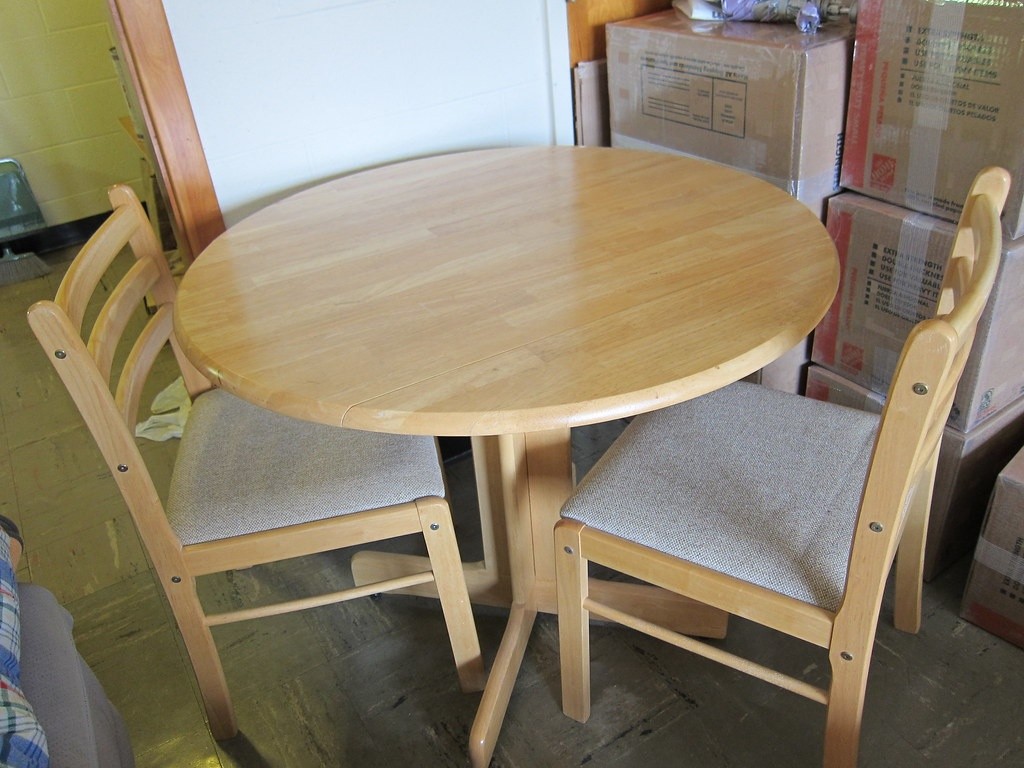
[[839, 0, 1024, 239], [959, 444, 1024, 651], [809, 189, 1024, 433], [802, 364, 1024, 570], [605, 9, 858, 397]]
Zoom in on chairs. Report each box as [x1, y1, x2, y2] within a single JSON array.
[[25, 184, 487, 739], [554, 164, 1013, 768]]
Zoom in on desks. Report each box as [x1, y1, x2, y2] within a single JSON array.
[[170, 144, 843, 768]]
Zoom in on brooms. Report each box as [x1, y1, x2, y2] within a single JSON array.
[[0, 244, 55, 288]]
